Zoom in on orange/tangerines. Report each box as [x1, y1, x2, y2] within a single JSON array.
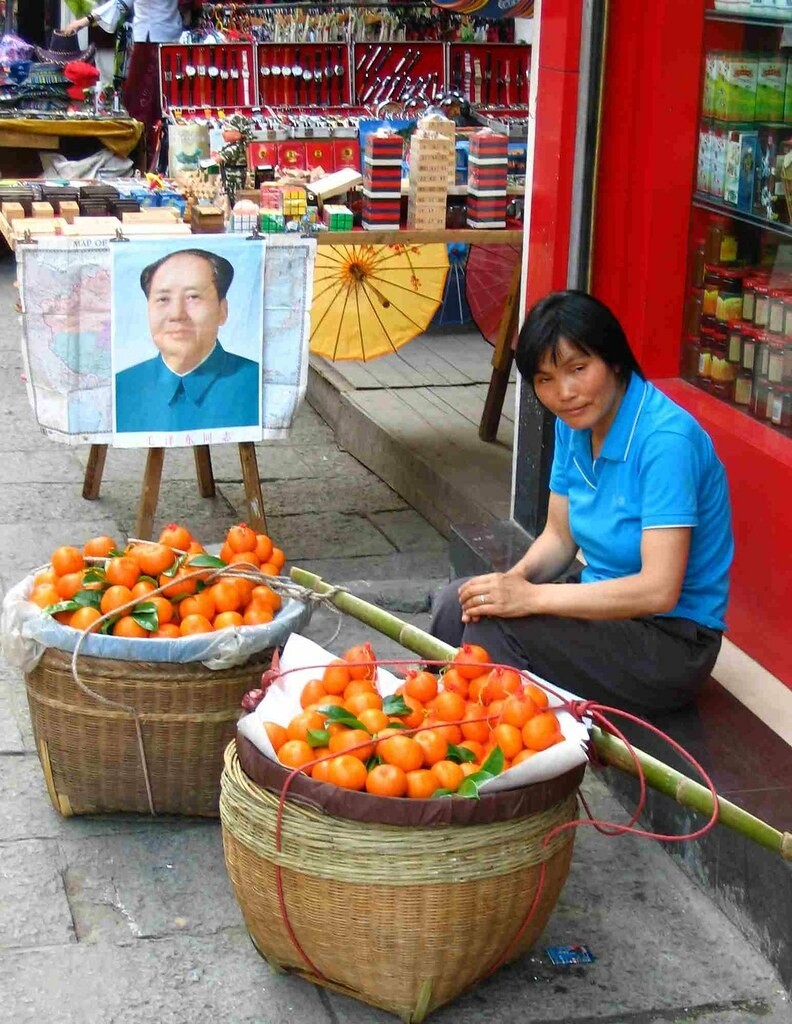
[[29, 524, 284, 640], [265, 647, 568, 800]]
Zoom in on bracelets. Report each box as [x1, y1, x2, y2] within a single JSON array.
[[87, 14, 95, 24]]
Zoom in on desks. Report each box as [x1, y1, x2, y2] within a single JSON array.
[[0, 118, 147, 158], [0, 178, 523, 540]]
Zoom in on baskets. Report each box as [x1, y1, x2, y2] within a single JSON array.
[[26, 646, 312, 817], [219, 738, 588, 1024]]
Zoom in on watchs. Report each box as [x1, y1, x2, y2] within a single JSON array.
[[165, 48, 344, 111]]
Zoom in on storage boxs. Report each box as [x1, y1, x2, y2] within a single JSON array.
[[697, 51, 792, 212]]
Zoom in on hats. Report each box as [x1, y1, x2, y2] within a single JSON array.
[[0, 29, 95, 120]]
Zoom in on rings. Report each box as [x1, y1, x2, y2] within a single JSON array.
[[481, 595, 486, 604]]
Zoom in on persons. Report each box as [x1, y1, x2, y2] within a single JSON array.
[[64, 1, 182, 171], [115, 250, 259, 432], [427, 291, 737, 715]]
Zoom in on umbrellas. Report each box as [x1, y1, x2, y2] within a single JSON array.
[[310, 242, 523, 364]]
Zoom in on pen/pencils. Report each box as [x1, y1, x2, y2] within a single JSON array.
[[356, 44, 438, 105]]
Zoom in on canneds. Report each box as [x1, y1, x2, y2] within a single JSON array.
[[684, 213, 792, 431]]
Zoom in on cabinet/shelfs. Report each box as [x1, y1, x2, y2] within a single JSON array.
[[691, 9, 792, 238]]
